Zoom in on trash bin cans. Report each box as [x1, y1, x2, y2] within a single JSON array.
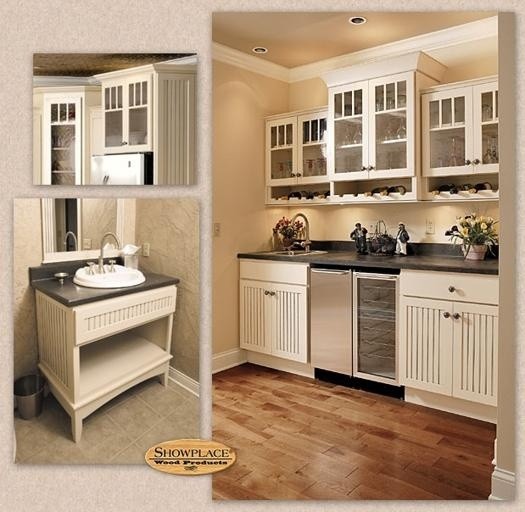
[[14, 374, 47, 420]]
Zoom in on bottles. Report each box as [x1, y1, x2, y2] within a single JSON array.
[[273, 156, 327, 179], [430, 181, 493, 197], [365, 184, 406, 197], [275, 190, 330, 201]]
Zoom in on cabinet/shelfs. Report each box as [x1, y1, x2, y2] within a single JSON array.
[[264, 106, 330, 205], [398, 271, 500, 408], [354, 269, 397, 388], [307, 265, 354, 381], [239, 257, 311, 385], [417, 75, 504, 201], [33, 282, 176, 444], [92, 62, 199, 153], [327, 48, 450, 201], [33, 86, 99, 185]]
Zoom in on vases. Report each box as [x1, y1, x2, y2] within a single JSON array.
[[460, 242, 489, 263], [282, 233, 294, 248]]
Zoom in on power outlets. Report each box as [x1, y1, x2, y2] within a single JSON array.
[[426, 220, 435, 235]]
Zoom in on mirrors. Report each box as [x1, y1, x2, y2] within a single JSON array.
[[38, 196, 123, 263]]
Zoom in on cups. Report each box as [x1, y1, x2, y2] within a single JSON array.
[[124, 254, 140, 269]]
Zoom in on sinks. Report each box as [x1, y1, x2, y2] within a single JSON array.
[[257, 250, 328, 257], [73, 264, 146, 289]]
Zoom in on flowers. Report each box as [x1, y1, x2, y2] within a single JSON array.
[[446, 205, 503, 262], [273, 216, 303, 237]]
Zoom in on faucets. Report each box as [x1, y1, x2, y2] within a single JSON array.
[[287, 213, 312, 253], [63, 231, 78, 251], [97, 232, 122, 274]]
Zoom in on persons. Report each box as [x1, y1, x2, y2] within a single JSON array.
[[394, 223, 410, 256], [350, 222, 369, 255]]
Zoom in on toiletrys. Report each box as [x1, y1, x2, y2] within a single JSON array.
[[102, 242, 118, 257], [119, 244, 141, 270]]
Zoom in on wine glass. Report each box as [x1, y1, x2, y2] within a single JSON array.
[[340, 114, 407, 146], [447, 133, 498, 168]]
